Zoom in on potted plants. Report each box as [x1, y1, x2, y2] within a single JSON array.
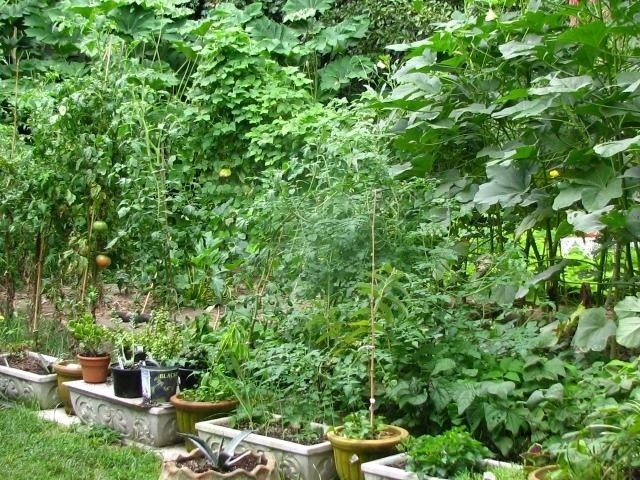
[[0, 190, 639, 480]]
